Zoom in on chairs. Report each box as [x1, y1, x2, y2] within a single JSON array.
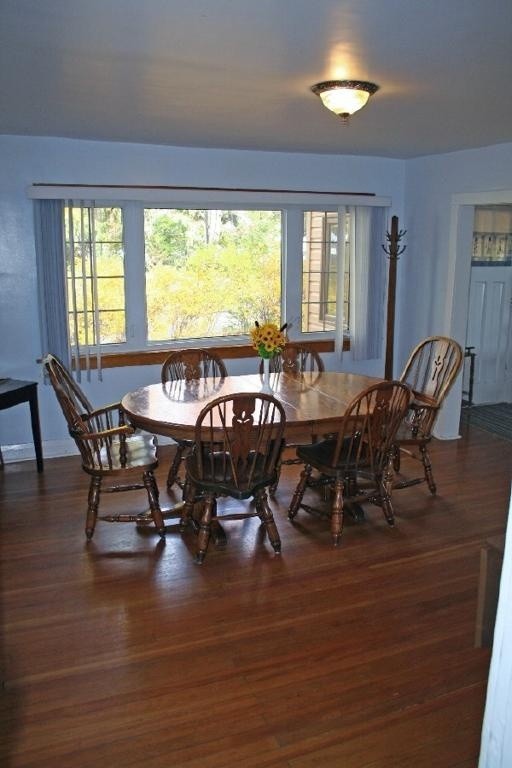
[[44, 353, 166, 544], [180, 392, 287, 566], [161, 347, 228, 490], [362, 335, 462, 514], [288, 380, 410, 548], [259, 342, 325, 499]]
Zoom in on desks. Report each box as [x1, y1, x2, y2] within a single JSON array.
[[1, 378, 43, 472], [119, 370, 415, 547]]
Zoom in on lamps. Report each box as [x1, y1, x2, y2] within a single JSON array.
[[310, 79, 379, 128]]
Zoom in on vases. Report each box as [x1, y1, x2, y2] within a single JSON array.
[[259, 358, 274, 396]]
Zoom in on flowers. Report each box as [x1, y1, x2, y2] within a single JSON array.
[[250, 321, 288, 359]]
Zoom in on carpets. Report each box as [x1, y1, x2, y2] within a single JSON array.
[[460, 401, 512, 443]]
